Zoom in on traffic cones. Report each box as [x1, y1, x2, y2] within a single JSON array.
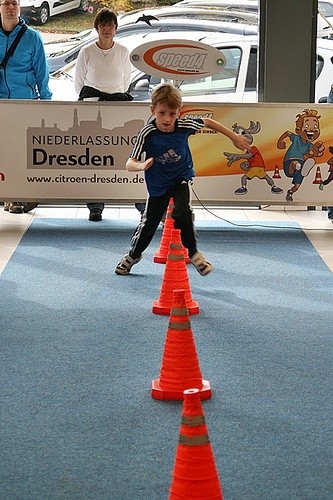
[[153, 197, 191, 264], [166, 387, 225, 500], [151, 289, 212, 402], [151, 229, 200, 315]]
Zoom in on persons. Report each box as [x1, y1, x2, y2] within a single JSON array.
[[74, 7, 147, 222], [113, 83, 252, 276], [0, 0, 52, 213]]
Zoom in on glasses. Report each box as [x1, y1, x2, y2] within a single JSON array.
[[0, 2, 18, 6], [97, 23, 116, 28]]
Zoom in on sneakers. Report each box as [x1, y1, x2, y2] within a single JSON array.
[[190, 252, 211, 276], [115, 253, 142, 275]]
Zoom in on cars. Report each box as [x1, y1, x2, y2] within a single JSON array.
[[128, 34, 333, 103], [18, 0, 89, 25], [44, 0, 333, 100]]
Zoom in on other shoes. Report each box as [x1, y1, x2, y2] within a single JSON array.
[[89, 209, 102, 221]]
